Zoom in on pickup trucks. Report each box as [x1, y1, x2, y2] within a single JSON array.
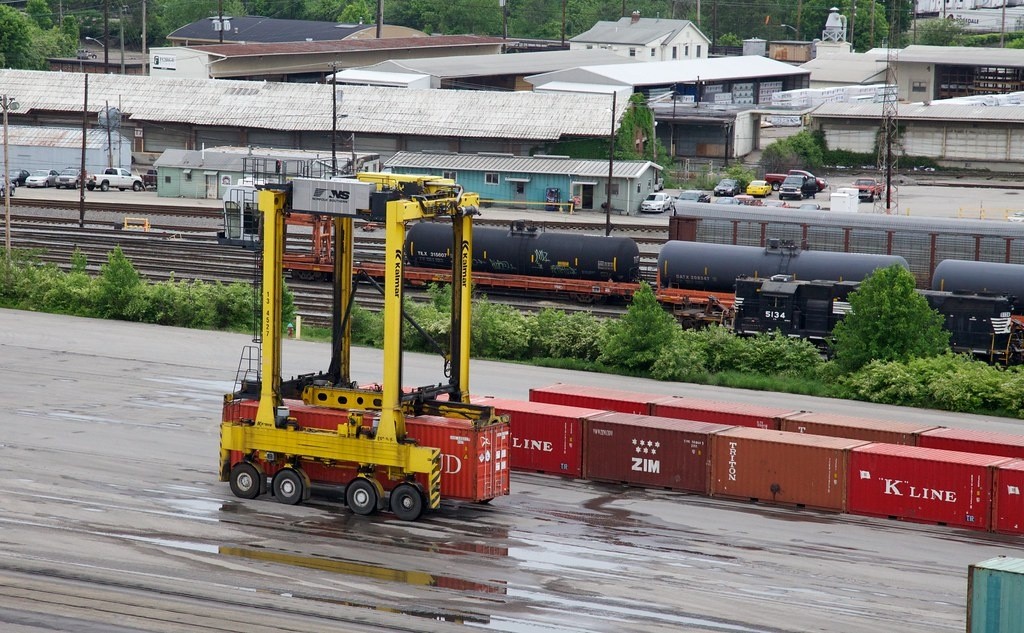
[[85, 167, 143, 192], [766, 169, 829, 192], [850, 177, 886, 202]]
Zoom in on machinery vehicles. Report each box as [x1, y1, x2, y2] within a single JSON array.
[[216, 170, 514, 522]]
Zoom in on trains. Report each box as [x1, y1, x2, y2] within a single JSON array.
[[277, 214, 1024, 374]]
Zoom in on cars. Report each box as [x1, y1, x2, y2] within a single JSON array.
[[713, 178, 742, 197], [76, 48, 98, 60], [716, 197, 741, 205], [0, 177, 15, 199], [674, 189, 703, 202], [24, 168, 60, 188], [640, 193, 674, 213], [5, 169, 30, 187], [746, 179, 773, 198]]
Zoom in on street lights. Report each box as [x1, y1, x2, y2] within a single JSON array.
[[326, 59, 342, 177], [0, 94, 19, 291]]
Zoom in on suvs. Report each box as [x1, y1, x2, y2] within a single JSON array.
[[54, 167, 91, 190], [778, 175, 817, 201]]
